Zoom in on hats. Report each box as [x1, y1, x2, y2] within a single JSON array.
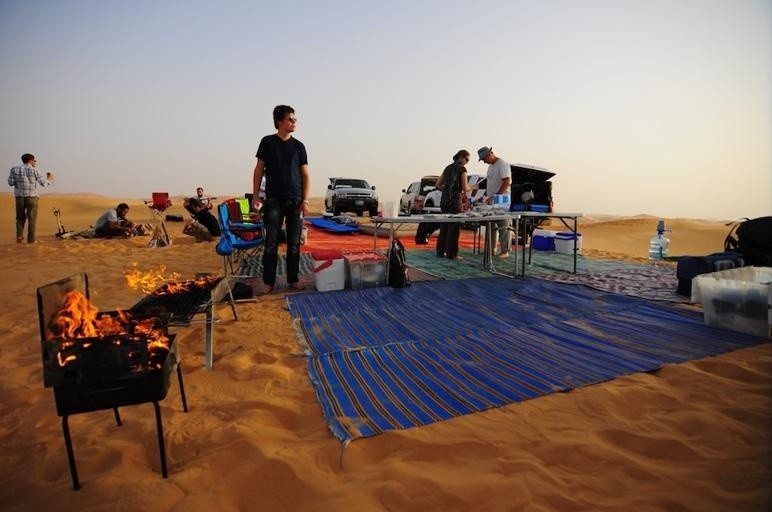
[[477, 146, 492, 161]]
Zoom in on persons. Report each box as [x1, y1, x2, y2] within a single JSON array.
[[95, 203, 136, 239], [7, 153, 54, 244], [258, 168, 267, 201], [478, 147, 512, 258], [435, 150, 478, 261], [251, 105, 310, 295], [182, 201, 222, 243], [193, 187, 213, 211]]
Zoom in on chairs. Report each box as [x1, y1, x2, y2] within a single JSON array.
[[143, 191, 171, 247], [217, 193, 267, 281]]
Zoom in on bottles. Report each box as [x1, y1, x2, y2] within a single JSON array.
[[254, 201, 263, 211]]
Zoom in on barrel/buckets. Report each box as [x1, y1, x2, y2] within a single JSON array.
[[647, 218, 673, 260], [477, 224, 501, 255]]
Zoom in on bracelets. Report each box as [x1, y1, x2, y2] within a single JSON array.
[[480, 195, 486, 200], [252, 194, 258, 199], [302, 199, 310, 205]]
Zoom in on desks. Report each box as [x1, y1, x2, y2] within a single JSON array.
[[370, 216, 522, 286], [507, 209, 582, 273]]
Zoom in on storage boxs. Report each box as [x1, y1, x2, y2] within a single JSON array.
[[532, 228, 554, 251], [344, 250, 388, 289], [691, 268, 772, 335], [309, 249, 345, 292], [554, 232, 581, 255]]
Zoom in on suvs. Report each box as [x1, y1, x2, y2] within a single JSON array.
[[398, 163, 556, 237], [325, 177, 379, 217]]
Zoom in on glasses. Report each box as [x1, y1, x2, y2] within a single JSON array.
[[465, 157, 468, 161]]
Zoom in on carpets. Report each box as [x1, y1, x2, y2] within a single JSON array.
[[240, 249, 772, 443]]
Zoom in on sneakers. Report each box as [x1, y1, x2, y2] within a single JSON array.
[[289, 280, 304, 290], [255, 284, 276, 296]]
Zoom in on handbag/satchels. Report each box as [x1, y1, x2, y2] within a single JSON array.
[[216, 238, 233, 256], [385, 240, 410, 288]]
